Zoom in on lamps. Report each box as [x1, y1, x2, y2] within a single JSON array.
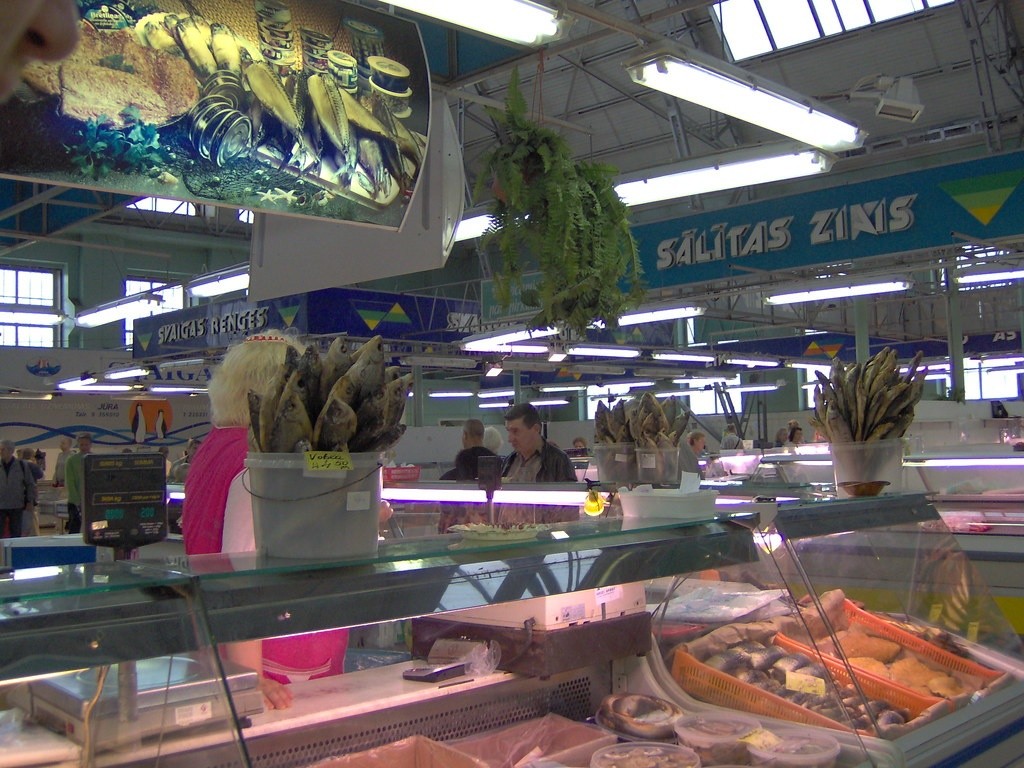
[[51, 0, 1024, 410]]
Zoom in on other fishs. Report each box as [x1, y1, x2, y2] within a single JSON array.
[[807, 346, 929, 477], [593, 391, 690, 484], [247, 335, 415, 453]]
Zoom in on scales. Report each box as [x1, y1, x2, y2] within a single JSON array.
[[23, 449, 265, 751]]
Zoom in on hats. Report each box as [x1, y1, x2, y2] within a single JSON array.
[[482, 427, 502, 453]]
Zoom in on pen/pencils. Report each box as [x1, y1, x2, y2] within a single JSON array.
[[437, 678, 475, 689]]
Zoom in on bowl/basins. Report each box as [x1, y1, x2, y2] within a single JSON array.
[[590, 690, 842, 768], [837, 480, 891, 497]]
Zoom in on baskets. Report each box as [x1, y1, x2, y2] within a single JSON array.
[[671, 634, 952, 737], [777, 598, 1001, 719]]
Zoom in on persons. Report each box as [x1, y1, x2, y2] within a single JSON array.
[[182, 328, 394, 710], [787, 419, 802, 443], [690, 431, 705, 453], [0, 440, 39, 540], [184, 439, 201, 464], [775, 428, 788, 447], [720, 424, 743, 449], [482, 426, 501, 454], [455, 419, 503, 524], [52, 437, 76, 488], [159, 446, 169, 458], [573, 437, 587, 449], [23, 448, 44, 537], [494, 403, 580, 523], [64, 433, 91, 534]]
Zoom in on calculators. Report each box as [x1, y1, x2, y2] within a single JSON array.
[[403, 662, 466, 683]]
